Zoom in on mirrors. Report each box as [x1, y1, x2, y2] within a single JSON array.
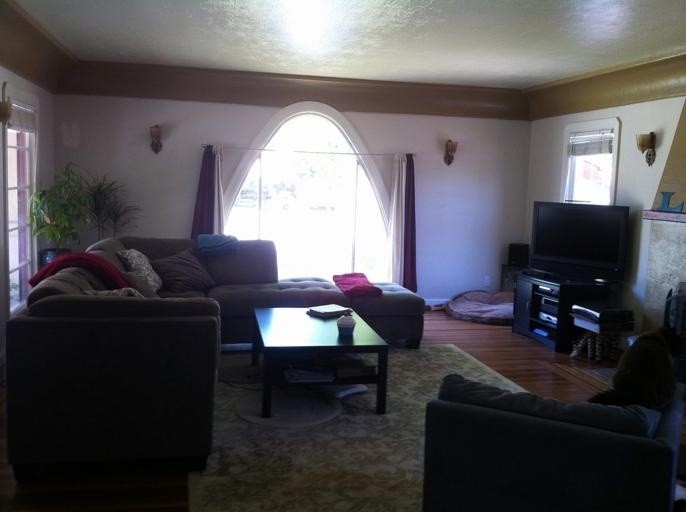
[[558, 117, 622, 205]]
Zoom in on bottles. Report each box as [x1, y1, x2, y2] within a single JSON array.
[[337, 312, 356, 336]]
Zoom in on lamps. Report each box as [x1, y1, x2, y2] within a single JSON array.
[[150, 125, 162, 154], [444, 140, 457, 166], [635, 131, 656, 167]]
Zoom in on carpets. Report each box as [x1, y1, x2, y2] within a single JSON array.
[[185, 342, 532, 512]]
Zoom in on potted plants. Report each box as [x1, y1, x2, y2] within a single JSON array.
[[30, 161, 95, 269]]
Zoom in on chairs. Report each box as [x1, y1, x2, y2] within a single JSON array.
[[421, 329, 685, 511]]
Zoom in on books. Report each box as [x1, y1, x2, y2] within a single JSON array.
[[306, 302, 353, 320]]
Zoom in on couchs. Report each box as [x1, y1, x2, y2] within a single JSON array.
[[5, 235, 424, 483]]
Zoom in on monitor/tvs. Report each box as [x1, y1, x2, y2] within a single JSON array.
[[528, 200, 631, 282]]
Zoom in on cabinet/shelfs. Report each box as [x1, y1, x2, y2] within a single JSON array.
[[511, 269, 623, 354]]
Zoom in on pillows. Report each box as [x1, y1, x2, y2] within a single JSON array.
[[82, 247, 217, 297]]
